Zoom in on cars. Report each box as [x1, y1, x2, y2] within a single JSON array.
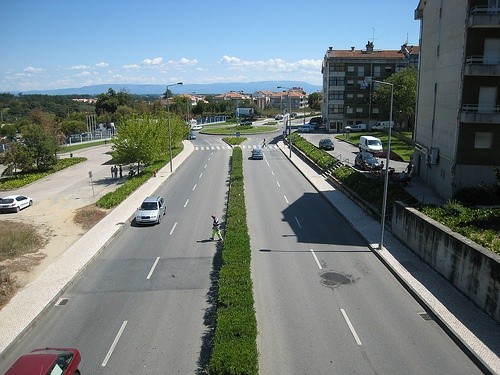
[[188, 132, 196, 140], [318, 139, 335, 150], [297, 121, 319, 132], [4, 347, 81, 375], [192, 125, 203, 130], [0, 195, 33, 213], [349, 125, 366, 132], [251, 148, 264, 160], [355, 152, 380, 170]]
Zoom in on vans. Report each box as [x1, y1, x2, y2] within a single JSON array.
[[371, 120, 395, 132], [358, 135, 383, 153]]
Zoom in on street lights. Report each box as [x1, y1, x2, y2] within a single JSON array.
[[364, 76, 394, 250], [277, 86, 292, 157], [166, 81, 183, 172]]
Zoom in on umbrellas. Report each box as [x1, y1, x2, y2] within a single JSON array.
[[373, 151, 405, 162]]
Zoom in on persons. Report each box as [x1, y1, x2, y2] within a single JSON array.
[[127, 167, 134, 178], [111, 167, 114, 178], [375, 161, 384, 171], [262, 139, 267, 148], [119, 165, 122, 178], [71, 153, 73, 157], [210, 215, 222, 240], [114, 166, 118, 178], [398, 171, 406, 180], [104, 140, 107, 145], [405, 162, 412, 175]]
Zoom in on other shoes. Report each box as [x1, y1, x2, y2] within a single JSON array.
[[218, 239, 222, 241], [210, 238, 214, 240]]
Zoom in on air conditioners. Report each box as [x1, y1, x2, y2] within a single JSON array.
[[428, 147, 438, 165]]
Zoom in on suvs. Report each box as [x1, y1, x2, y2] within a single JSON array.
[[135, 196, 167, 225], [240, 121, 253, 126]]
